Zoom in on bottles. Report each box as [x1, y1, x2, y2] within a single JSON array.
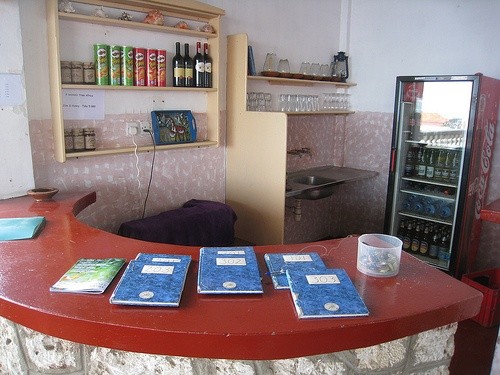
[[395, 83, 474, 265], [454, 93, 487, 279], [193, 42, 205, 87], [183, 43, 194, 87], [204, 43, 212, 88], [172, 42, 185, 87]]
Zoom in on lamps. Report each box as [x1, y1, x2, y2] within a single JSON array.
[[334, 51, 349, 82]]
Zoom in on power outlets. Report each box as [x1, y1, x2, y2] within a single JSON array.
[[125, 122, 140, 136], [139, 122, 150, 135]]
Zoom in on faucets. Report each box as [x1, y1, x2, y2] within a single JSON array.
[[287, 149, 303, 159], [295, 147, 314, 158]]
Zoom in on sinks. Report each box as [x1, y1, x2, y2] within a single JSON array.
[[295, 176, 342, 200], [285, 184, 301, 193]]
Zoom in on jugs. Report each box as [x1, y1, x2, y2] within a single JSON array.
[[330, 52, 349, 82]]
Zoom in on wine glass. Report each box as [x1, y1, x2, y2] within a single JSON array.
[[279, 95, 320, 112], [246, 92, 273, 112], [322, 92, 350, 111]]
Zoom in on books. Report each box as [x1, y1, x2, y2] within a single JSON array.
[[197, 246, 264, 294], [109, 253, 192, 307], [0, 216, 47, 241], [49, 257, 127, 295], [285, 267, 370, 319], [264, 252, 327, 289]]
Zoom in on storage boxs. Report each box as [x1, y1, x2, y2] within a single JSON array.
[[461, 268, 500, 328]]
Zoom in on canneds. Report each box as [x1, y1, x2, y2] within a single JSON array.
[[61, 60, 96, 85], [65, 128, 96, 153]]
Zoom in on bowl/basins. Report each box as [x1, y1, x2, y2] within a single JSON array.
[[356, 232, 403, 277]]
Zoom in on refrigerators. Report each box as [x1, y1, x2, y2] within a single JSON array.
[[381, 72, 500, 283]]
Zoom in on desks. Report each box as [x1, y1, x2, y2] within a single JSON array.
[[480, 199, 500, 224]]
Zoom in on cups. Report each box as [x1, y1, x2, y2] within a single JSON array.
[[263, 52, 290, 74], [300, 62, 332, 79]]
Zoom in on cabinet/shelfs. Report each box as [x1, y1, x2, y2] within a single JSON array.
[[247, 75, 357, 115], [45, 0, 225, 164]]
